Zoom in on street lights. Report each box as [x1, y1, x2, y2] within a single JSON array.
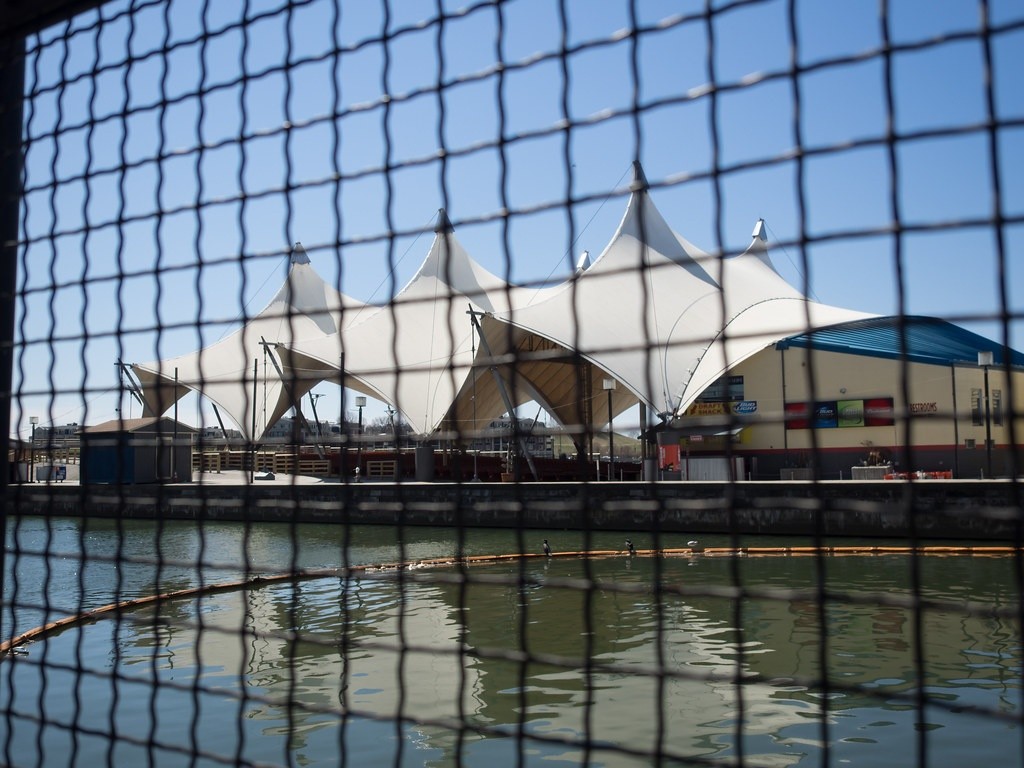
[[29, 416, 38, 484], [355, 396, 366, 483], [977, 351, 994, 480]]
